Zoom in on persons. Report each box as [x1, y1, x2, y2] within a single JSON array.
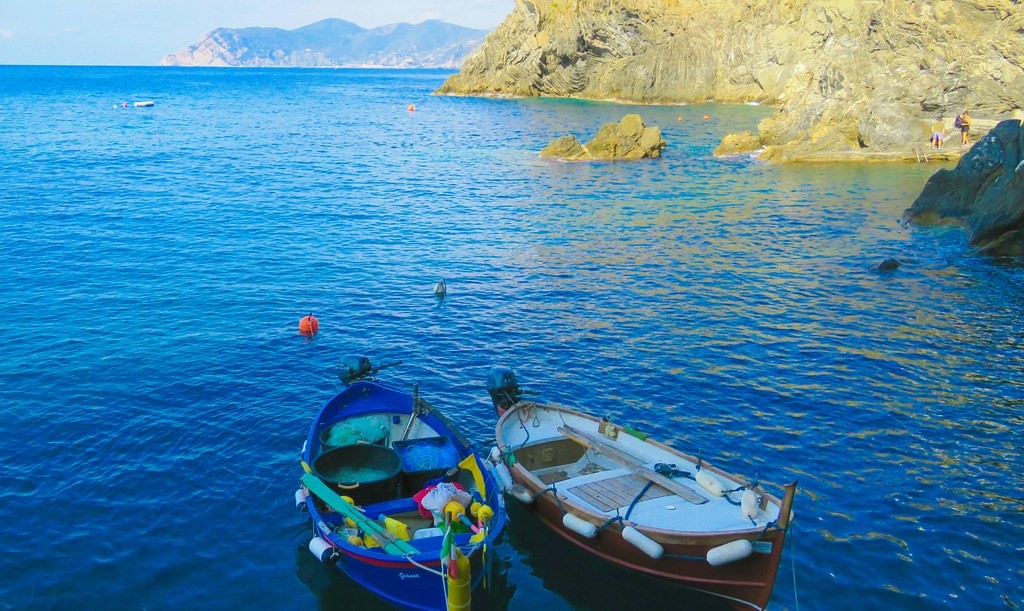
[[931, 116, 946, 150], [955, 111, 971, 145]]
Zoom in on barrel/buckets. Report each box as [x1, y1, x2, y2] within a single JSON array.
[[310, 421, 402, 506]]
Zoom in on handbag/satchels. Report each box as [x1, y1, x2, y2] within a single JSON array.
[[421, 483, 472, 526]]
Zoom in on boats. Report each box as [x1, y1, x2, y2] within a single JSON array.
[[134, 101, 154, 108], [294, 354, 510, 611], [747, 101, 761, 106], [484, 366, 801, 610]]
